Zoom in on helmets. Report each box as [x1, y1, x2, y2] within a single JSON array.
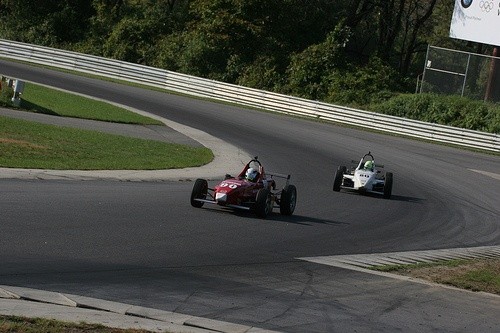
[[245, 168, 259, 182], [364, 160, 373, 171]]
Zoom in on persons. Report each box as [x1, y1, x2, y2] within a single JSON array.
[[245, 169, 259, 181], [363, 161, 374, 171]]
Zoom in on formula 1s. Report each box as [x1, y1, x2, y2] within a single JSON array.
[[333, 152, 394, 199], [190, 156, 297, 219]]
